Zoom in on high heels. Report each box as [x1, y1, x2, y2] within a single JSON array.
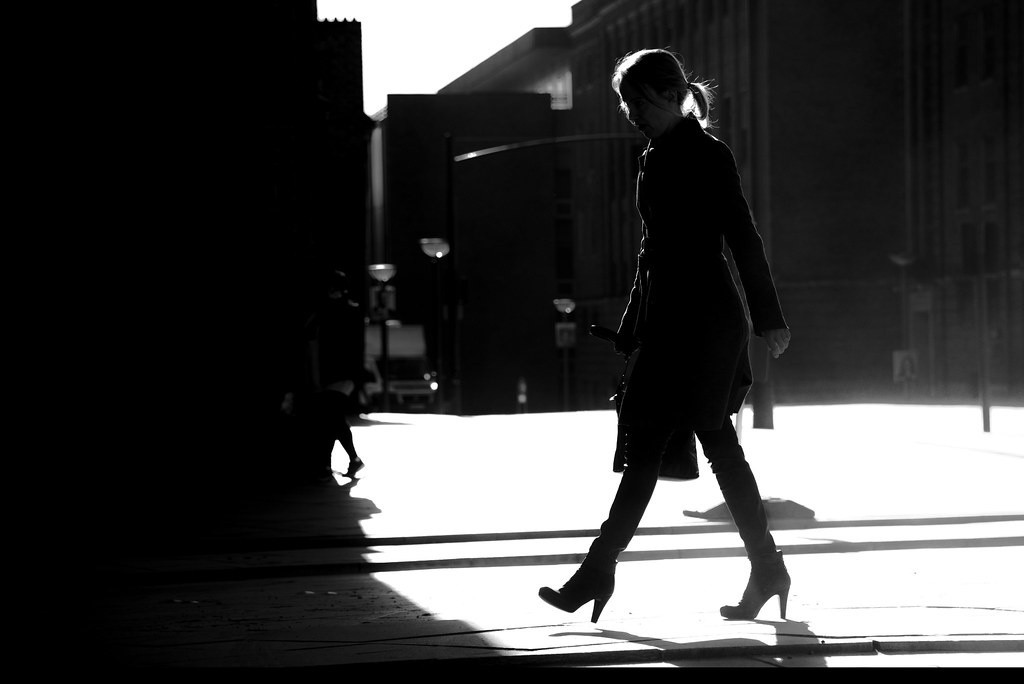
[[538, 556, 619, 623], [720, 550, 791, 620]]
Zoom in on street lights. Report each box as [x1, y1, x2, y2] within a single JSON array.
[[554, 288, 576, 411], [368, 262, 396, 413], [421, 237, 459, 412]]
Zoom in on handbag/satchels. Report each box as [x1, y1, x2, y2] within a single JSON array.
[[589, 322, 701, 482]]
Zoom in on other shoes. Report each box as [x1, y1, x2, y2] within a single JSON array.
[[343, 456, 364, 477]]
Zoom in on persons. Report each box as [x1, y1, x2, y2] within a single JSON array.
[[317, 420, 364, 482], [537, 46, 791, 622]]
